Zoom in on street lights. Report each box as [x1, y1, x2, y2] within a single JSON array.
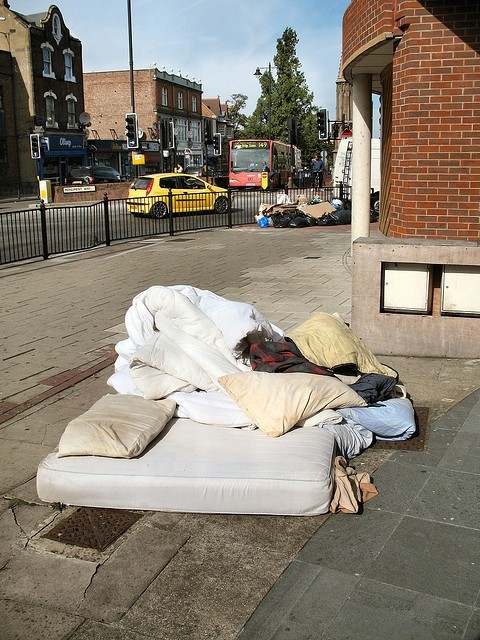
[[254, 61, 271, 141]]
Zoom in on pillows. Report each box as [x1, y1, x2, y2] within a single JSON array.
[[218, 372, 364, 438], [56, 393, 178, 460]]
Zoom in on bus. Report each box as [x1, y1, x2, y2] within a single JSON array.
[[228, 137, 303, 191]]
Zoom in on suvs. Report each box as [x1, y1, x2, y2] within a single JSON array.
[[64, 165, 126, 186]]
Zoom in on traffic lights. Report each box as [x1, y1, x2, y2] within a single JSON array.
[[168, 122, 175, 149], [30, 134, 42, 160], [124, 113, 139, 149], [213, 133, 221, 156], [316, 109, 327, 138]]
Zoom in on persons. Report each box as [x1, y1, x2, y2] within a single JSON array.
[[177, 162, 183, 172], [310, 155, 326, 191], [259, 161, 274, 190]]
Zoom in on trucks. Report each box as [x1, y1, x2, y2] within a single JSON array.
[[331, 135, 381, 214]]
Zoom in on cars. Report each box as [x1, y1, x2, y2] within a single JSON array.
[[183, 165, 206, 177], [127, 172, 234, 218]]
